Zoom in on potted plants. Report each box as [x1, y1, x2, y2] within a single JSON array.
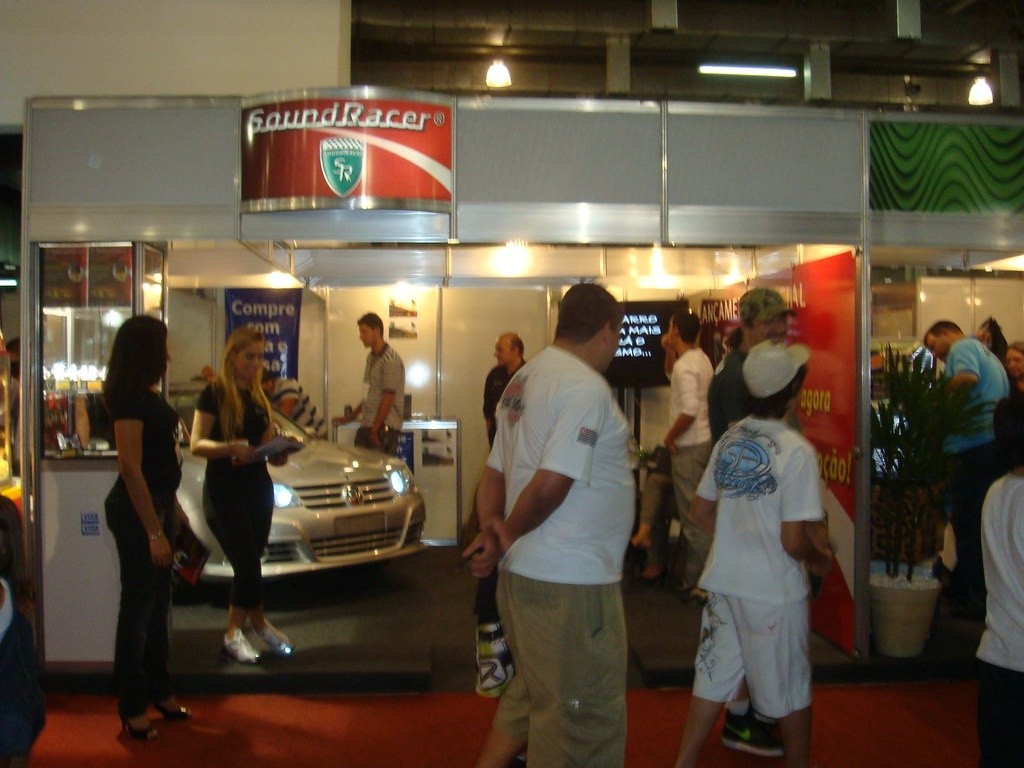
[[866, 341, 998, 658]]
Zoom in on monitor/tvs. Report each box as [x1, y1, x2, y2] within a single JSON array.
[[603, 300, 689, 389]]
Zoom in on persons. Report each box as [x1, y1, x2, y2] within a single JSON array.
[[678, 340, 838, 767], [462, 282, 640, 766], [483, 333, 531, 448], [201, 365, 328, 441], [662, 306, 715, 604], [706, 288, 801, 760], [627, 474, 679, 586], [189, 328, 295, 663], [921, 314, 1024, 768], [5, 338, 51, 476], [332, 312, 406, 457], [104, 317, 191, 741]]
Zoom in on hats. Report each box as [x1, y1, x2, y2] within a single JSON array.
[[743, 341, 810, 398], [739, 288, 797, 322]]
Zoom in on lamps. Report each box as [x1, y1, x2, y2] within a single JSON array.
[[485, 58, 512, 87], [968, 76, 993, 106]]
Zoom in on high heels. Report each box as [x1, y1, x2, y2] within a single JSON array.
[[119, 707, 158, 740], [154, 699, 192, 720]]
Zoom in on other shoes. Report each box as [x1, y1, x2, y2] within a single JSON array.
[[626, 540, 648, 554], [630, 565, 668, 588], [673, 587, 707, 608]]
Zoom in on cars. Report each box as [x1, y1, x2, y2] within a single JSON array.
[[88, 395, 427, 587]]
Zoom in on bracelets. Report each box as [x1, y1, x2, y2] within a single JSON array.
[[147, 530, 164, 542]]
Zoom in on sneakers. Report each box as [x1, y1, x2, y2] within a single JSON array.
[[223, 629, 262, 662], [721, 701, 783, 757], [254, 618, 291, 655]]
